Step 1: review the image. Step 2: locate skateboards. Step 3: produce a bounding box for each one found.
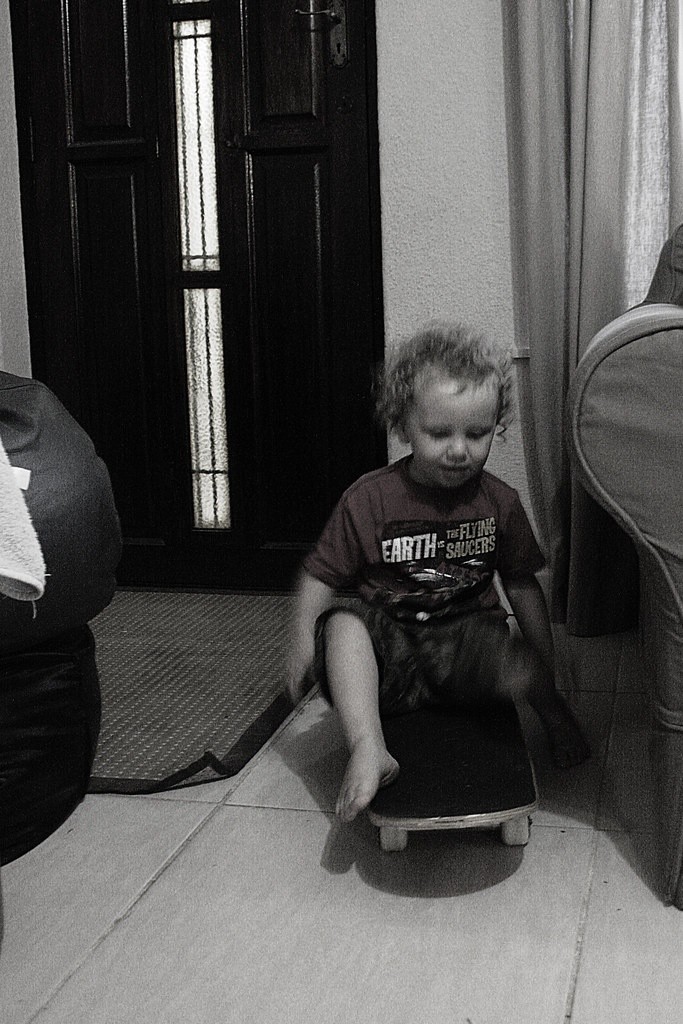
[365,677,543,858]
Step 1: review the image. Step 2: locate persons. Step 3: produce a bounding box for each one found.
[287,323,593,824]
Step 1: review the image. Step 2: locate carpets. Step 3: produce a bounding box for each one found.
[78,590,317,795]
